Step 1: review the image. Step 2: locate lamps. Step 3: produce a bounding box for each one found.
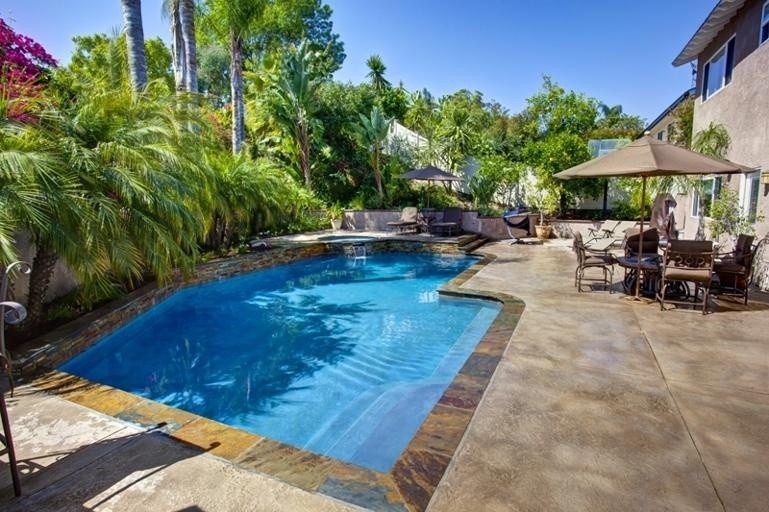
[761,171,769,185]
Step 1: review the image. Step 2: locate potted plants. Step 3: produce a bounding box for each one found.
[331,201,344,229]
[530,177,560,239]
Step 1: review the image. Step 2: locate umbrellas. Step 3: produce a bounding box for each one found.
[552,131,757,301]
[398,166,460,208]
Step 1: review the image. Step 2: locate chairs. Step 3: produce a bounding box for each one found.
[432,205,463,236]
[387,205,417,233]
[0,260,32,498]
[572,219,762,314]
